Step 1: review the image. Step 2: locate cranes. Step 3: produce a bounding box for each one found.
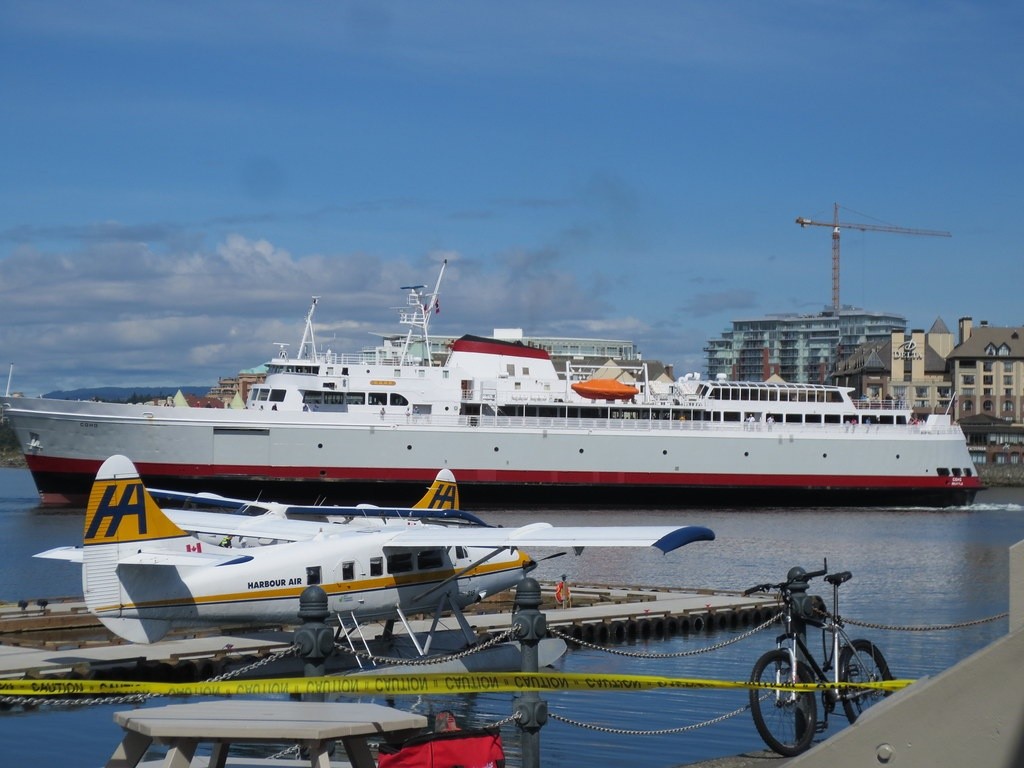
[796,203,952,313]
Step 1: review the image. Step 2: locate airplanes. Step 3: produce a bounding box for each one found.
[32,455,716,645]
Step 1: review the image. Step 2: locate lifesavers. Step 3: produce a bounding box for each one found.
[556,582,571,603]
[0,595,827,713]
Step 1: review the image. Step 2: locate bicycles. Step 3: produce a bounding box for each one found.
[740,557,898,756]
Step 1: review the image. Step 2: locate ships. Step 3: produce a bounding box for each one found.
[1,258,982,512]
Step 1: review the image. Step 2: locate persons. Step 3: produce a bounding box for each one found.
[218,536,233,549]
[679,415,685,429]
[845,392,961,434]
[745,413,776,431]
[92,397,422,425]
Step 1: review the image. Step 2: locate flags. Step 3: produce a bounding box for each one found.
[424,298,440,315]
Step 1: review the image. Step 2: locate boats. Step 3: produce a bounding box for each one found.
[572,378,640,399]
